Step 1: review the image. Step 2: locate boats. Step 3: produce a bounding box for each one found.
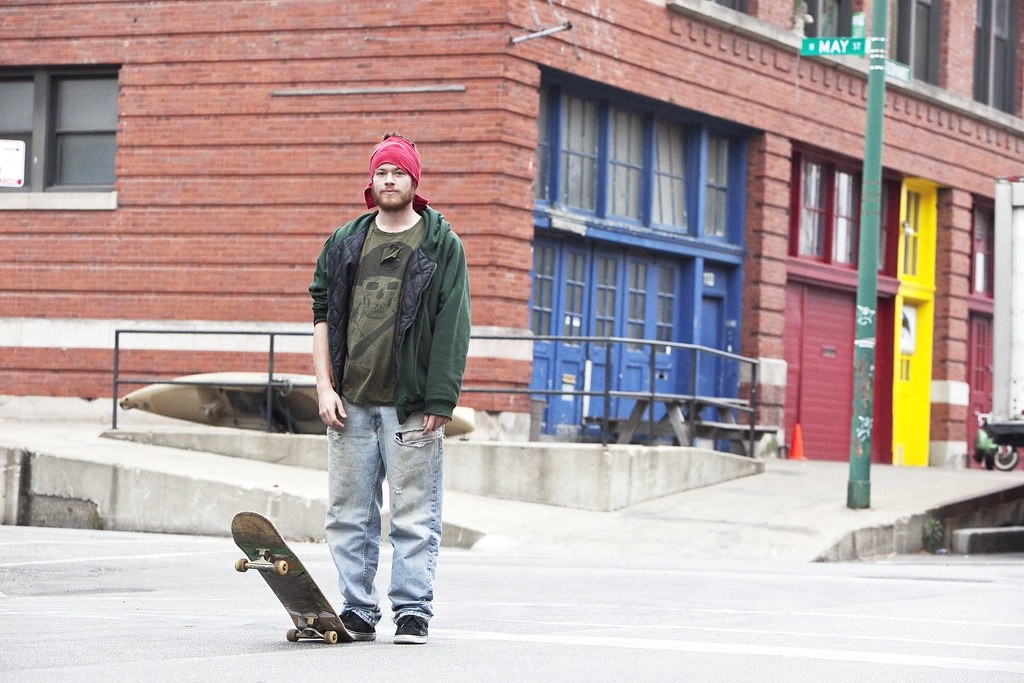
[118,371,476,438]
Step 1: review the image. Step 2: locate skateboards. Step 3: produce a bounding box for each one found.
[230,510,355,644]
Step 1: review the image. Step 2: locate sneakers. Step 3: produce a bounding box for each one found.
[337,610,376,641]
[393,615,429,644]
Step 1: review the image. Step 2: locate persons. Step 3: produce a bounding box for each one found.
[308,134,472,643]
